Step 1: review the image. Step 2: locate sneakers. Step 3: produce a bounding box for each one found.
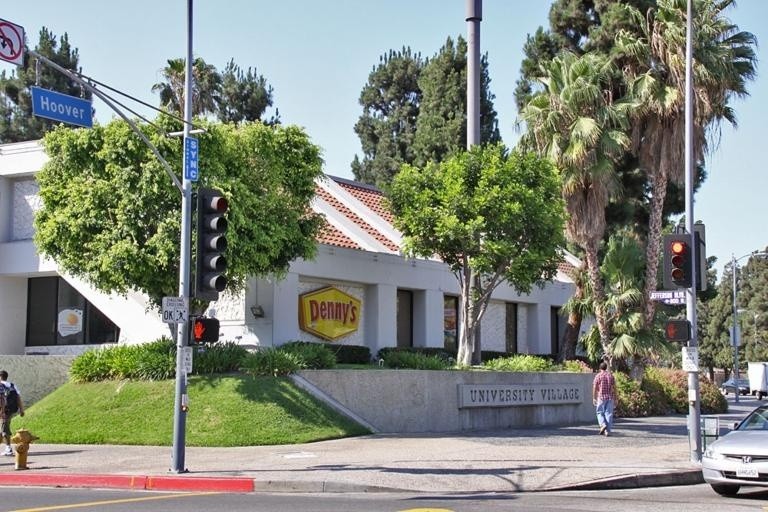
[2,449,13,455]
[599,424,610,436]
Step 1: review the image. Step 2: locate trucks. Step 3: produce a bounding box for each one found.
[748,362,768,400]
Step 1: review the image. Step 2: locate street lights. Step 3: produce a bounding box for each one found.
[730,252,766,402]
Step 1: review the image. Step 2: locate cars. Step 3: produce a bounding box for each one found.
[722,379,750,395]
[701,403,768,497]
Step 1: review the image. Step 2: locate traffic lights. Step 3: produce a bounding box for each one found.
[195,186,228,302]
[188,314,219,345]
[663,233,692,291]
[665,319,690,341]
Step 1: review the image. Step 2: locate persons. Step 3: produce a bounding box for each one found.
[592,362,619,437]
[1,370,25,456]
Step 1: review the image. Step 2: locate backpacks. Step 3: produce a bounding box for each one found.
[0,383,18,415]
